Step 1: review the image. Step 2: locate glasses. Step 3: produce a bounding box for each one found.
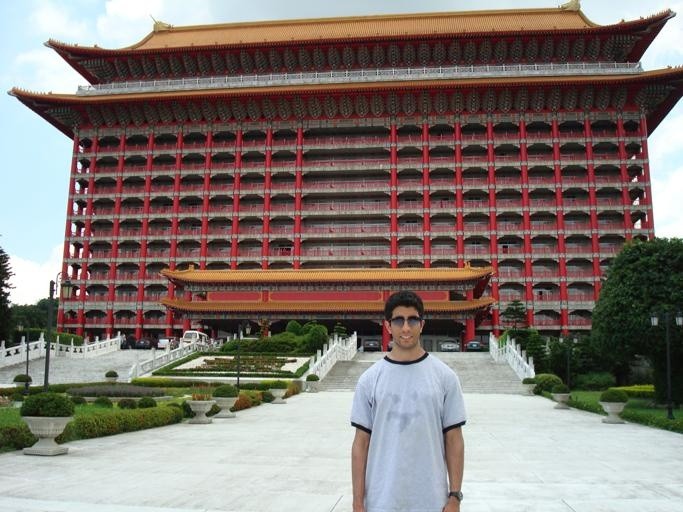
[387,314,422,328]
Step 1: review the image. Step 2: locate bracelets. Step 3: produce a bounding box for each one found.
[447,491,463,501]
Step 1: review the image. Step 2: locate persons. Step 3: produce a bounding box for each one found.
[349,291,465,512]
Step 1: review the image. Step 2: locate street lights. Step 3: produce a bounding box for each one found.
[557,323,581,390]
[17,316,31,398]
[234,320,250,393]
[42,269,71,393]
[650,308,682,419]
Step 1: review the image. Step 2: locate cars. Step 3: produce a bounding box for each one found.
[363,338,379,352]
[386,339,393,350]
[465,340,481,351]
[440,339,459,352]
[120,330,226,353]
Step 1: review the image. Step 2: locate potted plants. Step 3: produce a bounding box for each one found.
[104,370,119,382]
[306,374,320,392]
[550,382,572,409]
[19,389,76,457]
[185,376,241,424]
[598,388,630,424]
[13,374,34,388]
